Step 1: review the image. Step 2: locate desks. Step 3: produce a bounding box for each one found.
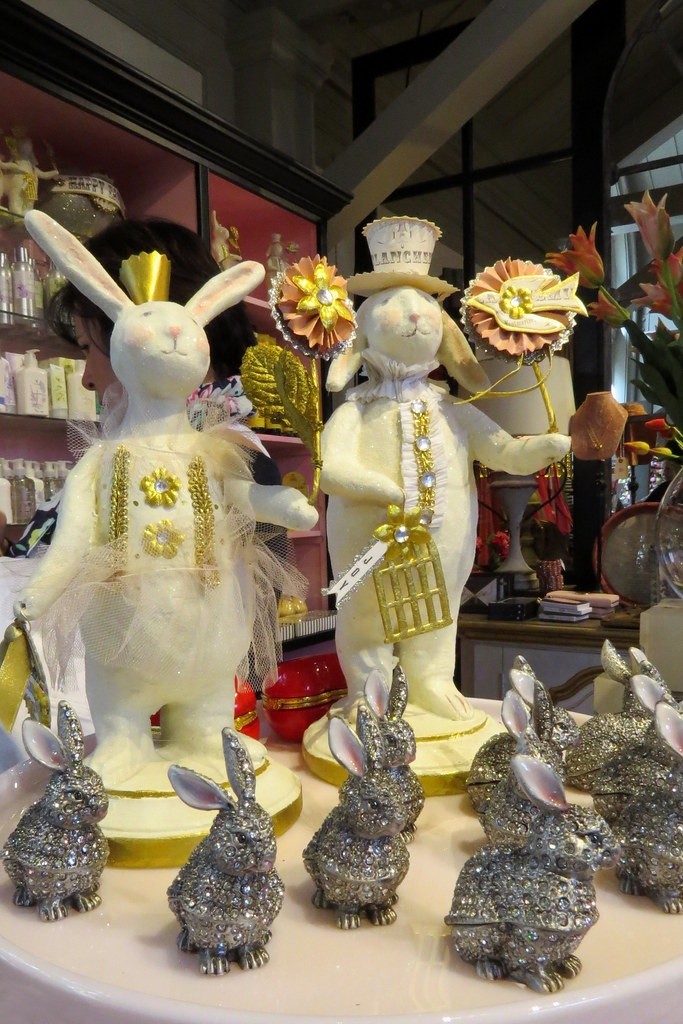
[456,613,641,716]
[0,697,683,1024]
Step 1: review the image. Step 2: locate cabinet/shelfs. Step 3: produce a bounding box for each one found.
[0,0,355,611]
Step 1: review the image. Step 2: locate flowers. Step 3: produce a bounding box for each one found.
[544,190,683,443]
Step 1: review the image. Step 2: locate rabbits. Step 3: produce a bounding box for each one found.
[443,651,625,996]
[1,698,111,923]
[167,726,286,976]
[300,663,426,932]
[559,636,683,917]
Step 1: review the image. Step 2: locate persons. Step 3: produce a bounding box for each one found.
[0,217,288,701]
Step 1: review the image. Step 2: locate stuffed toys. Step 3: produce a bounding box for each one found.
[24,210,571,786]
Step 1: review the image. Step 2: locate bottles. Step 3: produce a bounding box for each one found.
[66,360,96,421]
[0,246,68,339]
